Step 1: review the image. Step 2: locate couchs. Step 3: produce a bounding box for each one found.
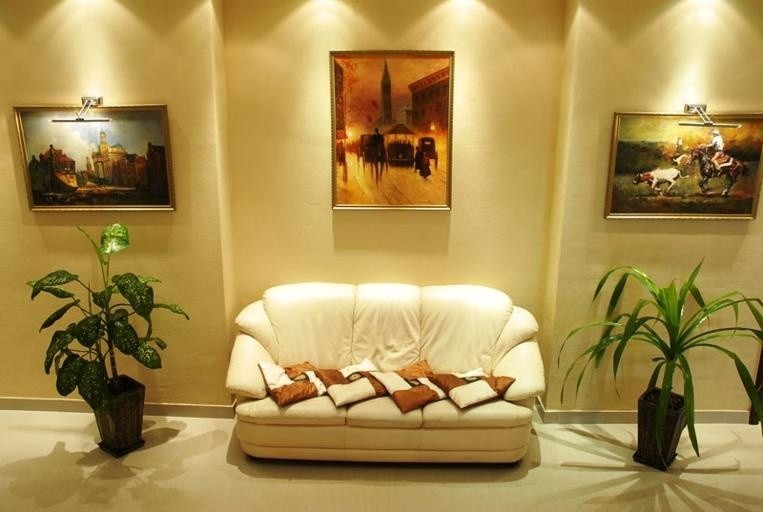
[226,281,546,466]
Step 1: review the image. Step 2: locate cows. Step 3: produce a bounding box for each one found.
[632,167,690,196]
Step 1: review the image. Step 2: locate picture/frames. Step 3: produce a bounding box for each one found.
[329,49,455,211]
[12,104,175,212]
[603,111,763,221]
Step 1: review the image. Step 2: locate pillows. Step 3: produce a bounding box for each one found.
[258,356,516,412]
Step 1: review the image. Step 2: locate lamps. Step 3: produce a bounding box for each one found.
[678,103,741,128]
[52,97,112,122]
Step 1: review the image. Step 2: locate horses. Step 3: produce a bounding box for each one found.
[368,141,385,187]
[687,147,750,198]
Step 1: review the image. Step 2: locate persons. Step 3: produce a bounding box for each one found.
[704,128,724,178]
[336,126,432,186]
[674,136,690,178]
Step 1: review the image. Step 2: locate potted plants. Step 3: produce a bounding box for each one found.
[557,255,763,472]
[25,223,189,458]
